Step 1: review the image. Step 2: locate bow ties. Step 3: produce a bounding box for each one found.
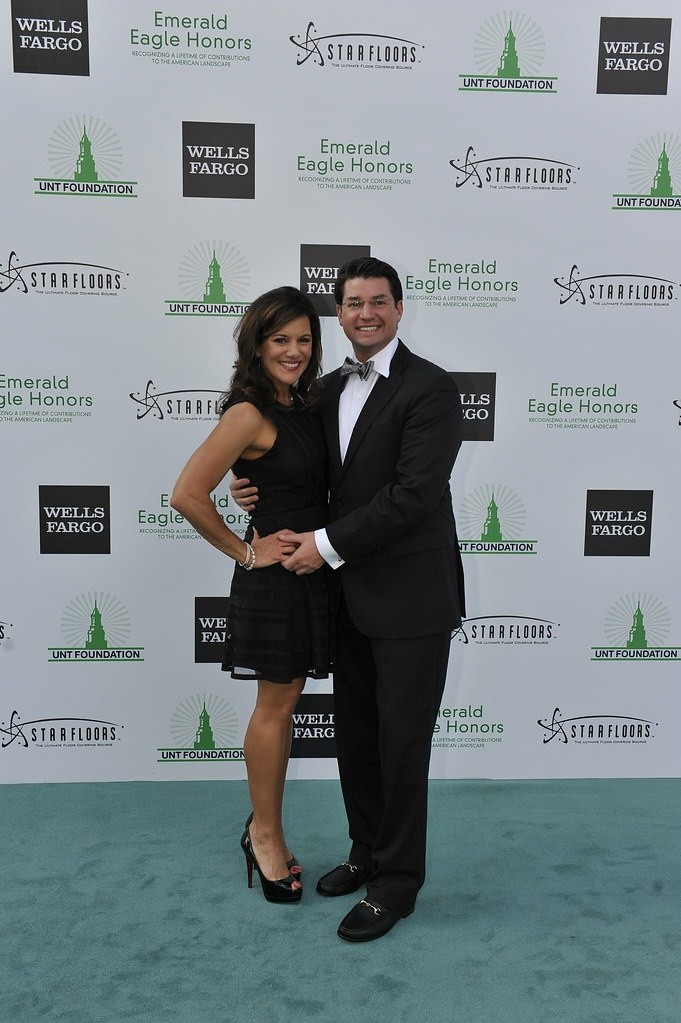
[340,356,374,381]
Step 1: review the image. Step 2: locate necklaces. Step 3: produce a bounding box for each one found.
[288,391,295,401]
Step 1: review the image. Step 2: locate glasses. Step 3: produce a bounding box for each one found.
[340,296,398,311]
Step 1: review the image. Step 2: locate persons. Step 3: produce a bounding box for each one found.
[169,288,339,906]
[231,256,467,943]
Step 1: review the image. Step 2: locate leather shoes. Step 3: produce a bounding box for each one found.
[315,859,416,941]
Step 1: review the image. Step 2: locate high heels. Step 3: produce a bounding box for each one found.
[240,808,304,906]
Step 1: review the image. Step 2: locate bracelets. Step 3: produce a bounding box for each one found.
[243,544,255,571]
[236,542,250,567]
